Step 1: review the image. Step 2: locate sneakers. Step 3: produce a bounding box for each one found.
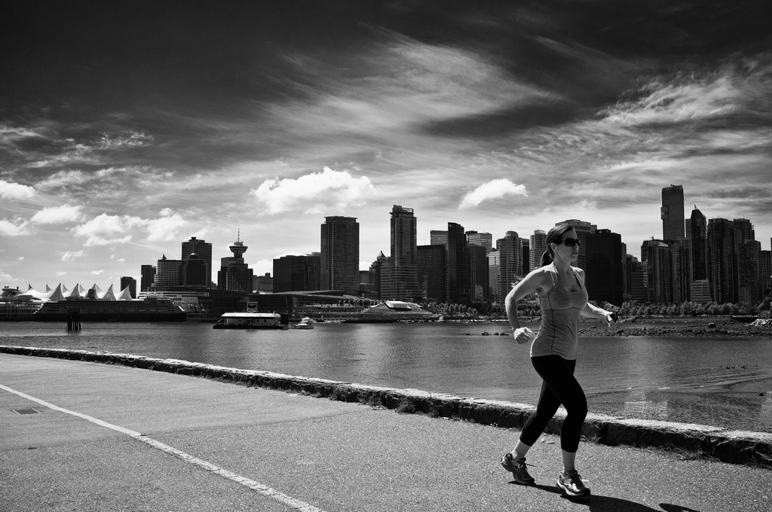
[500,453,534,485]
[556,470,590,496]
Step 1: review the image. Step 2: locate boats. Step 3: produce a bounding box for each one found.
[212,313,284,330]
[296,317,315,330]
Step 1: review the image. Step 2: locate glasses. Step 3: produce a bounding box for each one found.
[561,238,582,247]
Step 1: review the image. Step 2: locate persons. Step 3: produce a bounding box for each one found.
[501,223,617,498]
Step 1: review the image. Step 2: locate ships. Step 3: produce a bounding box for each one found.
[33,295,185,320]
